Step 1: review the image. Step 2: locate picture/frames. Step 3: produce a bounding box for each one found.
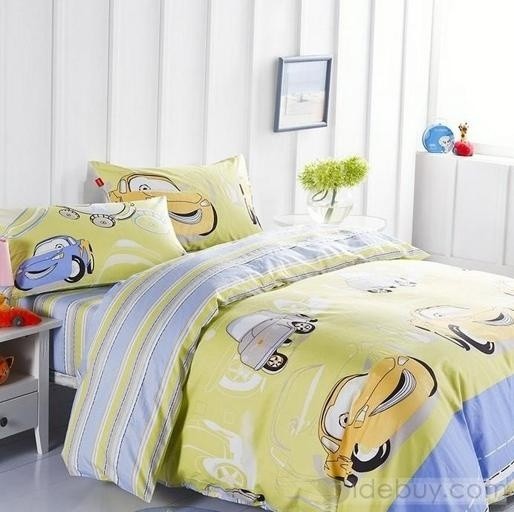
[272,54,331,135]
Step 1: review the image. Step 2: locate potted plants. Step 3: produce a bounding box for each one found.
[296,154,370,227]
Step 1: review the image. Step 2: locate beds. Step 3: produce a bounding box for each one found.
[0,207,513,494]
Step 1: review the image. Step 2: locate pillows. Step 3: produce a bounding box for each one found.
[0,154,267,300]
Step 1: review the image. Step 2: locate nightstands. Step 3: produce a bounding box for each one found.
[0,315,62,456]
